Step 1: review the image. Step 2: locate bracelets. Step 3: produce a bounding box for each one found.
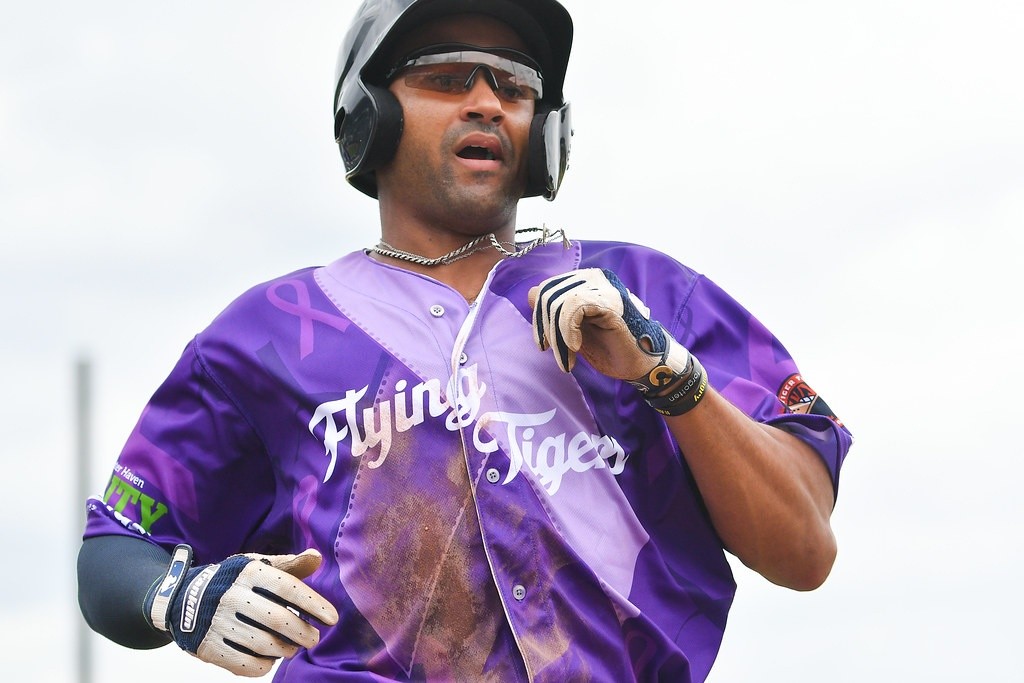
[645,354,708,416]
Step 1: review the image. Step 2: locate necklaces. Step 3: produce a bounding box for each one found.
[374,225,570,265]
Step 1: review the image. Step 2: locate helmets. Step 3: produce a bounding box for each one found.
[331,0,573,201]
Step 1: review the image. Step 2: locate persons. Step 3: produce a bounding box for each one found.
[76,1,853,683]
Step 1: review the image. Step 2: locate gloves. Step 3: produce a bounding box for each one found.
[527,266,693,397]
[139,540,341,677]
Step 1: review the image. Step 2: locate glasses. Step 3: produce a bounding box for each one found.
[380,42,547,101]
